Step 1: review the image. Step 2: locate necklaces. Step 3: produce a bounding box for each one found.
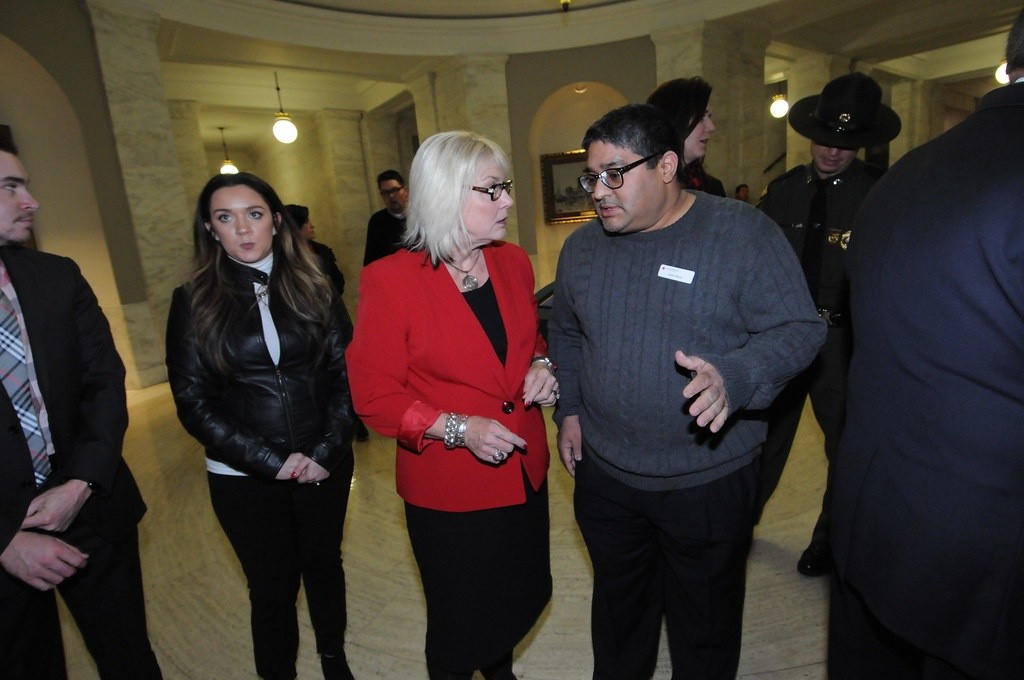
[440,248,481,292]
[256,288,267,299]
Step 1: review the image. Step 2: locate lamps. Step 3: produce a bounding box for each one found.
[270,70,299,144]
[995,60,1011,84]
[560,0,571,12]
[216,125,239,175]
[769,82,790,118]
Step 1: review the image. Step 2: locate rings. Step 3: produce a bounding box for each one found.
[493,450,505,462]
[551,390,560,399]
[314,481,320,485]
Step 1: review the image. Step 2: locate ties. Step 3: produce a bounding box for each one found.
[0,291,51,487]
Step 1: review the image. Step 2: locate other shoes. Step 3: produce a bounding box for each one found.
[355,418,369,441]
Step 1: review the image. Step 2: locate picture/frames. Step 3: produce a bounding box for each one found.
[538,147,602,227]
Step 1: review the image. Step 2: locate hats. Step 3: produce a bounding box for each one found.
[788,73,902,150]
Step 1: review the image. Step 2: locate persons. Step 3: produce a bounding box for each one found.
[647,75,728,201]
[734,183,749,203]
[342,131,561,680]
[164,172,363,680]
[823,5,1024,680]
[745,72,902,580]
[549,102,827,680]
[364,169,423,269]
[282,204,370,446]
[1,124,164,680]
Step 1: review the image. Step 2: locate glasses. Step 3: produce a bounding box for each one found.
[463,179,513,202]
[577,151,664,194]
[379,185,404,198]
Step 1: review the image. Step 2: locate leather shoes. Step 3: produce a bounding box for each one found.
[798,545,836,577]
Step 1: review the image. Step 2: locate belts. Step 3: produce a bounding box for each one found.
[815,309,852,325]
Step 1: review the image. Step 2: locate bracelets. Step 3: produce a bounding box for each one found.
[444,413,472,449]
[87,481,97,492]
[530,355,555,375]
